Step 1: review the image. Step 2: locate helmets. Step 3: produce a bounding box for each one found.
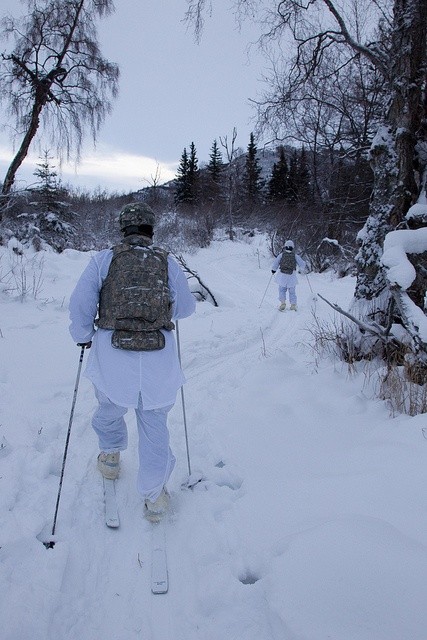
[284,240,295,250]
[116,202,155,233]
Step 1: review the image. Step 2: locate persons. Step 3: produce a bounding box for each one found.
[271,238,302,311]
[65,199,196,515]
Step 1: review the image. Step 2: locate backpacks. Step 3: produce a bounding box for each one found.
[279,250,296,275]
[89,244,174,351]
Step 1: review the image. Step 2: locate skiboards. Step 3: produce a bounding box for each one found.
[102,477,170,595]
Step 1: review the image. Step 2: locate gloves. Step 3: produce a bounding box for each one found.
[271,269,276,274]
[76,338,92,352]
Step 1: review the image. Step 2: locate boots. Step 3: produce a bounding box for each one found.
[96,451,121,482]
[144,487,173,521]
[290,305,298,312]
[278,301,287,311]
